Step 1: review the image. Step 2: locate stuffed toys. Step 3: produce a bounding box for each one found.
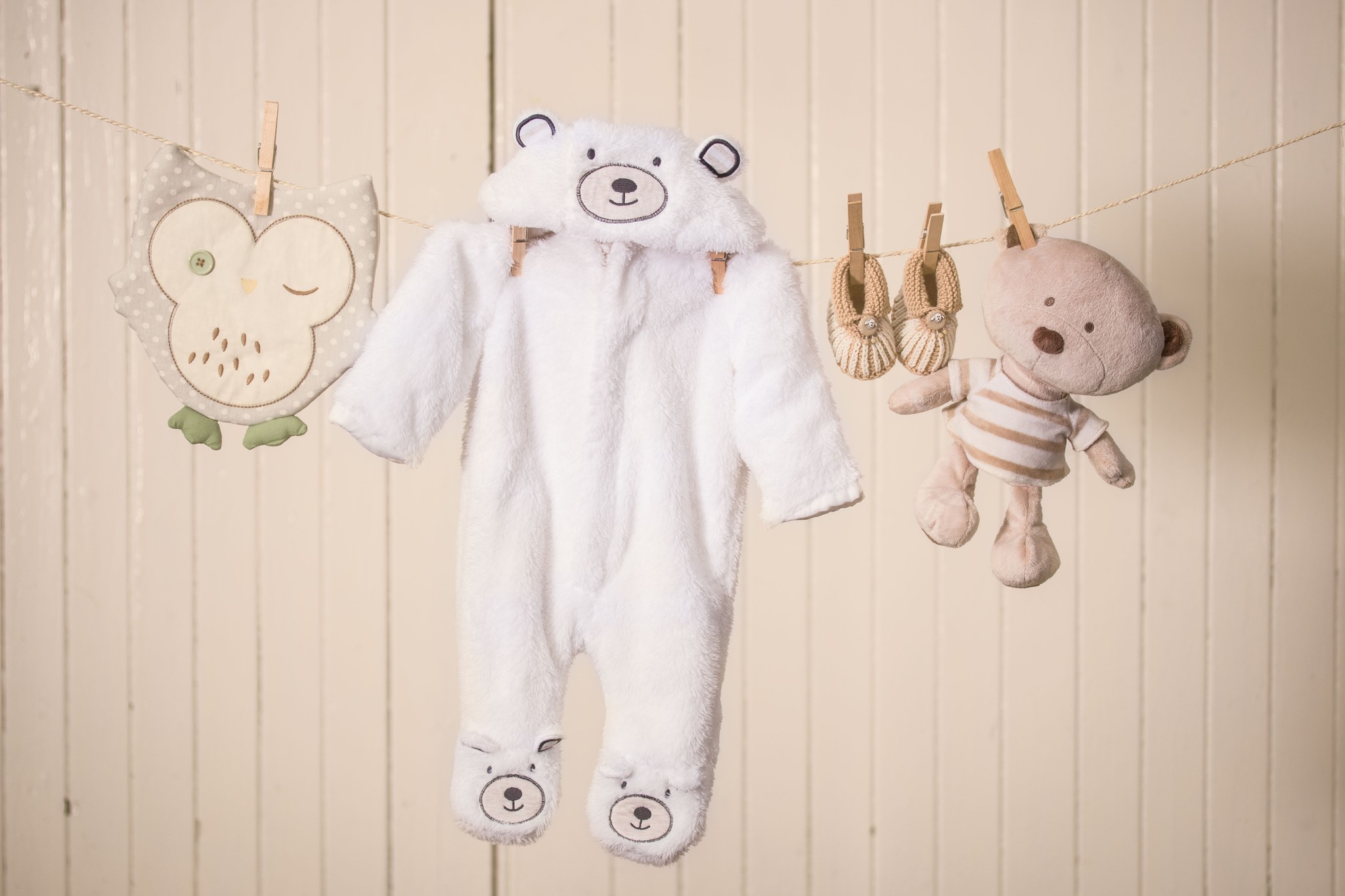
[889,219,1194,592]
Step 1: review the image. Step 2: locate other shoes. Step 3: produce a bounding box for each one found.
[891,249,964,376]
[826,253,897,381]
